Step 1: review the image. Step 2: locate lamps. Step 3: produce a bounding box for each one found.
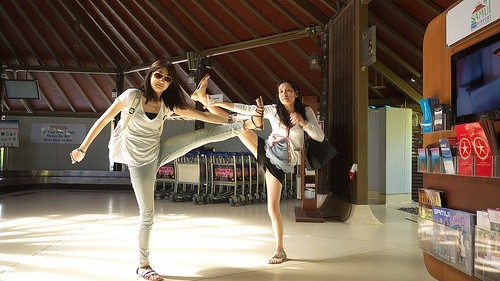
[188,71,195,85]
[310,51,320,70]
[112,88,117,98]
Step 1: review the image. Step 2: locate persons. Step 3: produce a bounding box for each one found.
[69,59,264,281]
[190,72,325,264]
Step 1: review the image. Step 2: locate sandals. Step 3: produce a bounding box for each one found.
[267,251,287,264]
[251,96,265,131]
[191,74,211,103]
[136,265,164,281]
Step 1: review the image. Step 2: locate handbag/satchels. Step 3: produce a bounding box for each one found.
[301,104,338,171]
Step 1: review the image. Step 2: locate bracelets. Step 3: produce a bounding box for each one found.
[227,116,232,123]
[77,147,86,154]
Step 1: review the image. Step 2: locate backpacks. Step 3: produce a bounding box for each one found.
[108,88,168,164]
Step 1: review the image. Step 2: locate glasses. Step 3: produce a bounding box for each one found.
[152,71,174,84]
